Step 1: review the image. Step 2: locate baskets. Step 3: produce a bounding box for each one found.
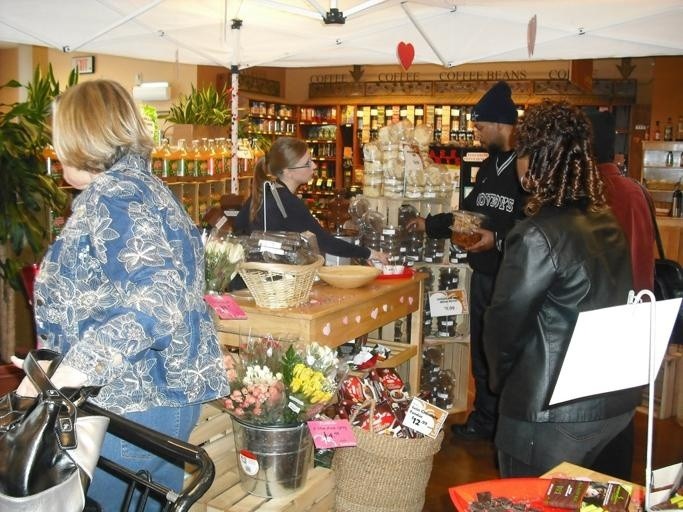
[236,255,326,311]
[331,396,445,511]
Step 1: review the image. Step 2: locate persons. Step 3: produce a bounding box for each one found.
[482,99,643,478]
[225,135,391,292]
[32,79,230,508]
[405,81,530,443]
[579,103,657,482]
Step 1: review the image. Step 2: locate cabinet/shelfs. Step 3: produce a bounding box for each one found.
[217,89,613,224]
[641,140,683,267]
[47,157,276,242]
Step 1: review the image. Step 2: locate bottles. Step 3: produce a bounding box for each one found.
[676,115,682,141]
[149,138,265,181]
[665,117,672,140]
[654,120,660,142]
[181,192,220,222]
[248,101,293,136]
[671,187,682,217]
[435,130,474,148]
[307,127,336,158]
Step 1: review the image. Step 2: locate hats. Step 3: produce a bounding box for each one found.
[583,107,616,162]
[470,80,518,124]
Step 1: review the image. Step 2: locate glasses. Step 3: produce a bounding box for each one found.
[278,152,312,174]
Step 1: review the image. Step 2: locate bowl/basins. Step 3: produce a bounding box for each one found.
[316,265,378,289]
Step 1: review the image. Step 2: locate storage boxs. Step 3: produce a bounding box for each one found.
[173,124,227,148]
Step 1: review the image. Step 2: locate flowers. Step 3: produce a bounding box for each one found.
[156,80,241,139]
[205,239,245,293]
[223,337,339,427]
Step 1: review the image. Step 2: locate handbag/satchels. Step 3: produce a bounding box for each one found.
[0,348,110,512]
[632,175,683,345]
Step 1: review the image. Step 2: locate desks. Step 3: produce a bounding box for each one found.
[214,272,429,467]
[538,462,646,512]
